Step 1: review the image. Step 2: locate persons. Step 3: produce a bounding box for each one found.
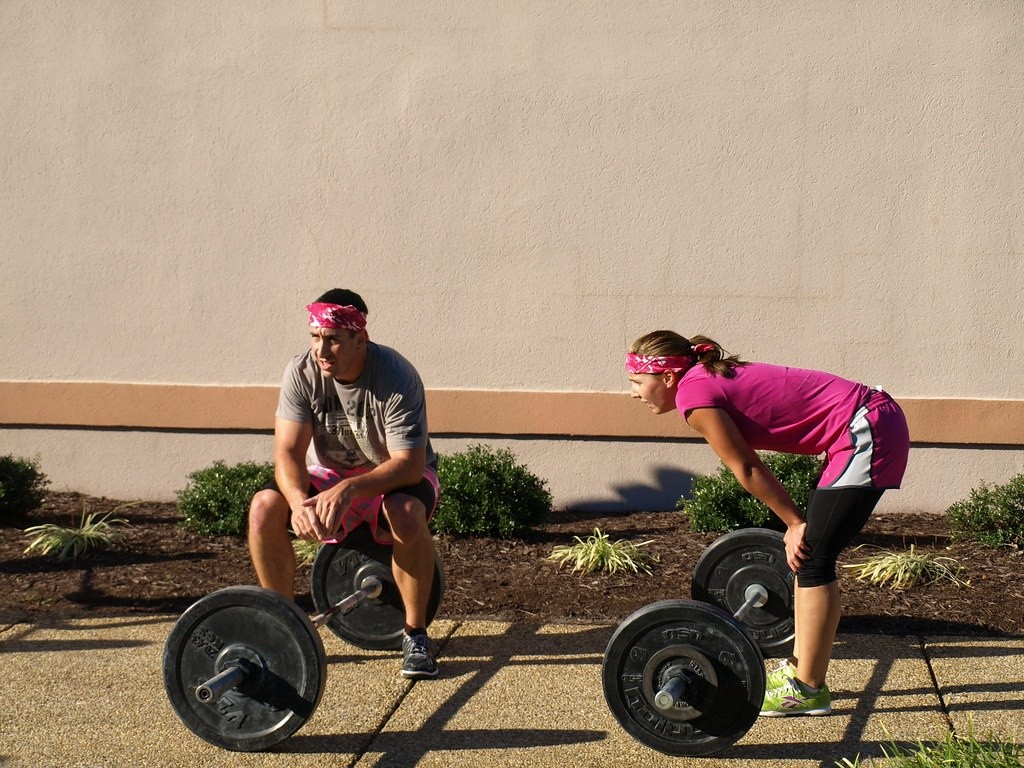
[248,286,444,680]
[626,329,912,717]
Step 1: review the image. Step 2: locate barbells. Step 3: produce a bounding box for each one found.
[158,521,448,755]
[598,522,816,760]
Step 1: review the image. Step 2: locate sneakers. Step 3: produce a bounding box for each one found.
[758,678,831,717]
[401,635,438,679]
[765,658,798,691]
